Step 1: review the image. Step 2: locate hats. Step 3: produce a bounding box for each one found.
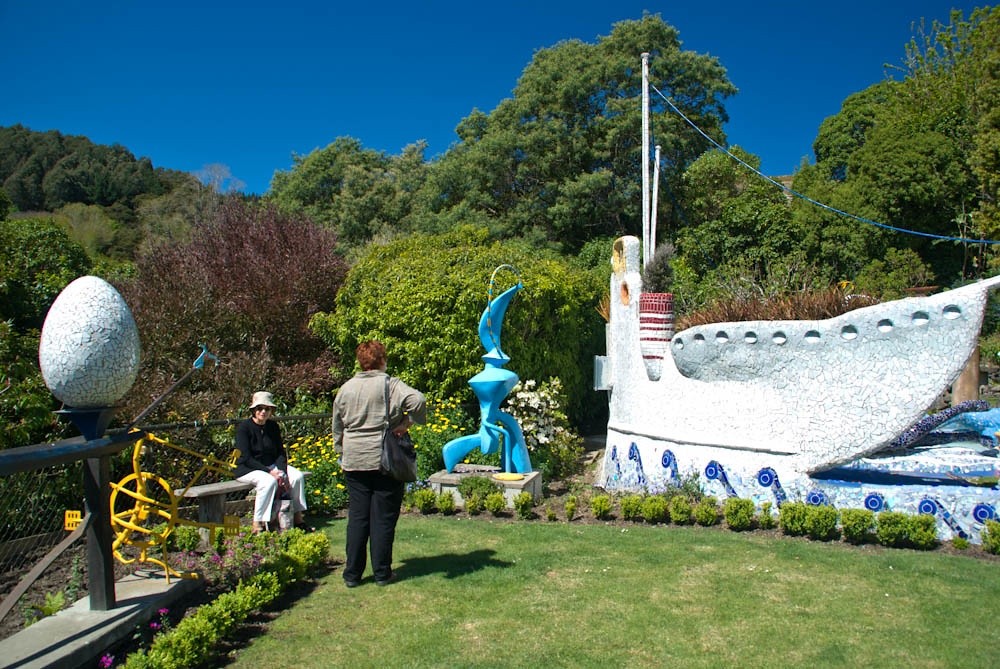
[249,391,278,409]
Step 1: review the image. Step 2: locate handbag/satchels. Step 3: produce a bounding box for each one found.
[381,376,417,482]
[268,476,293,532]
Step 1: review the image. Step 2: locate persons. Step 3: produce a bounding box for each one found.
[233,391,312,534]
[332,340,426,589]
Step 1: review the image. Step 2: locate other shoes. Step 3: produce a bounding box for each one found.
[375,573,400,586]
[294,522,315,533]
[344,578,362,588]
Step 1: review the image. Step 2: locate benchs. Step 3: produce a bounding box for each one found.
[174,472,311,523]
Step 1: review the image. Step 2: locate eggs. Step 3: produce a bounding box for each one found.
[39,275,142,409]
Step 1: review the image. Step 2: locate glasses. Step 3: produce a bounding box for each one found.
[256,407,271,412]
